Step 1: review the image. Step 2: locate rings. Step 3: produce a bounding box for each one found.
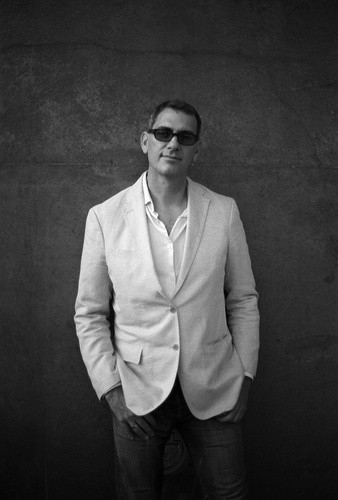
[131,424,137,430]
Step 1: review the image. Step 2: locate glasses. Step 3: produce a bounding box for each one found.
[147,127,200,146]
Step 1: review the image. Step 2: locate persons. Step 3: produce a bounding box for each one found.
[73,99,259,500]
